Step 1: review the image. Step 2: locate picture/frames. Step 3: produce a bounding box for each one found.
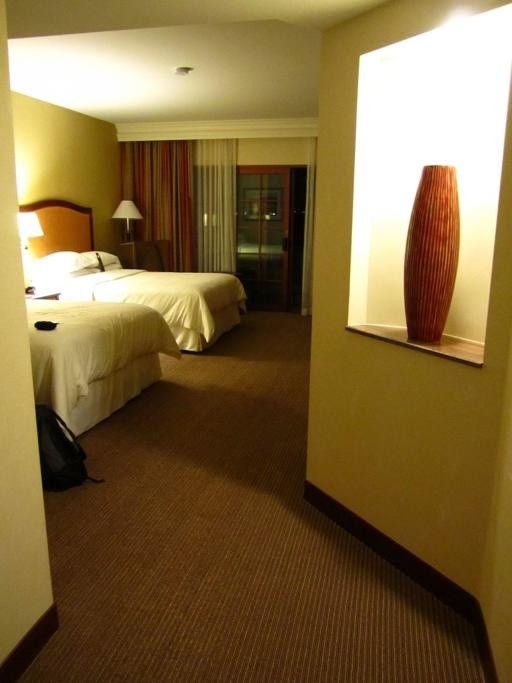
[243,187,284,223]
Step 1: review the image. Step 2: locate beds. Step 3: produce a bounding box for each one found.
[24,299,180,443]
[20,201,246,352]
[237,235,284,263]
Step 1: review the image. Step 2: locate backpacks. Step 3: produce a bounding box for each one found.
[35,401,105,492]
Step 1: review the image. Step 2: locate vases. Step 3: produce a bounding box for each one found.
[405,166,459,346]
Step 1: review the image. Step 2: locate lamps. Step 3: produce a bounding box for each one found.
[111,200,143,241]
[18,212,44,238]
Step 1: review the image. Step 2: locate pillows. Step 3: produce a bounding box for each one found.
[39,251,123,275]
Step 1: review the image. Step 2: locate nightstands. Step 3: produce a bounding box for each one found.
[24,288,60,300]
